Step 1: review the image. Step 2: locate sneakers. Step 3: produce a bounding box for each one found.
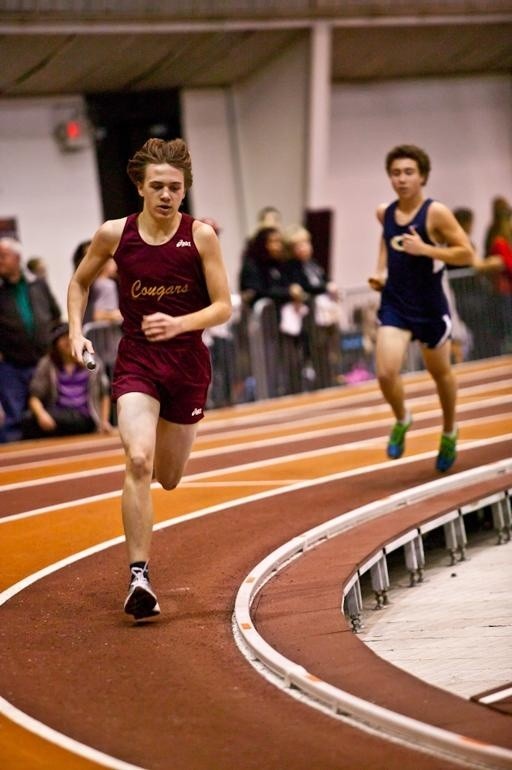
[434,425,460,473]
[124,567,162,622]
[386,415,412,461]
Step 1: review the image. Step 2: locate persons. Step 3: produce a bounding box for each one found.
[369,144,472,473]
[1,236,124,441]
[198,206,345,408]
[66,136,234,618]
[445,208,479,295]
[472,204,512,299]
